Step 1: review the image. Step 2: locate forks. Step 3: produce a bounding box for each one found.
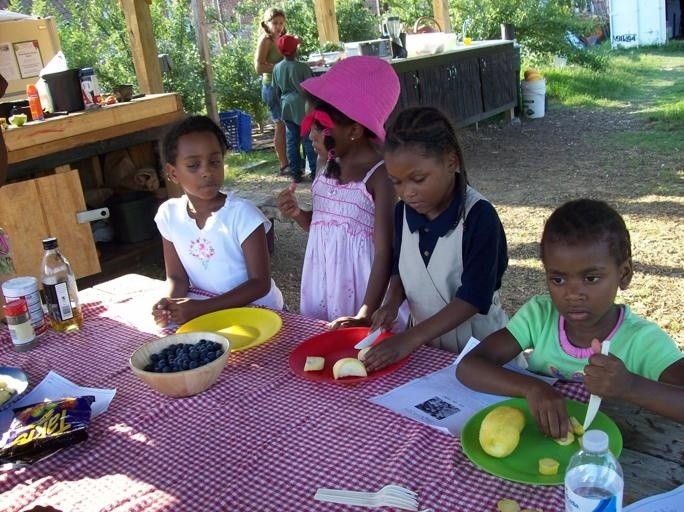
[313,485,419,512]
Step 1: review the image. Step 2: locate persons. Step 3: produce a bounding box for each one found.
[276,56,401,331]
[152,115,287,328]
[363,108,528,374]
[456,199,684,439]
[254,7,292,175]
[271,35,316,183]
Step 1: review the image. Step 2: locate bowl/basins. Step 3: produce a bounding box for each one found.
[128,332,231,398]
[8,113,27,126]
[113,85,132,102]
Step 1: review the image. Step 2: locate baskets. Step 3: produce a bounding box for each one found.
[219,109,253,153]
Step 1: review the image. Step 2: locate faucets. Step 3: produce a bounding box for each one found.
[411,16,442,33]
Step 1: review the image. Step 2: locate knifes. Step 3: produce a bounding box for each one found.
[582,341,609,430]
[354,325,386,350]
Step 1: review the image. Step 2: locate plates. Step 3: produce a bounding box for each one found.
[289,327,415,386]
[0,366,31,411]
[175,306,284,353]
[462,393,624,486]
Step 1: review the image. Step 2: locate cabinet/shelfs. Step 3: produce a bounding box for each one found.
[0,120,187,304]
[313,46,522,131]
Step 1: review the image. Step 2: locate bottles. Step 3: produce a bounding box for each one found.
[80,67,100,107]
[565,431,624,511]
[464,35,471,47]
[26,83,44,121]
[0,237,84,351]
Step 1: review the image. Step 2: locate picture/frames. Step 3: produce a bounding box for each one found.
[12,40,45,79]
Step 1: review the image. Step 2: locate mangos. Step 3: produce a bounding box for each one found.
[478,404,526,459]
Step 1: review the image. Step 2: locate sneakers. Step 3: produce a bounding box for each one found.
[280,164,292,176]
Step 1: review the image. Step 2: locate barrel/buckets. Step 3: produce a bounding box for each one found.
[41,68,86,113]
[520,77,547,120]
[103,190,157,244]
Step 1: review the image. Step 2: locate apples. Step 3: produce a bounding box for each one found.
[303,356,326,372]
[333,357,367,379]
[358,345,372,361]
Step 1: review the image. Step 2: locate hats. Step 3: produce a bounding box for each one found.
[299,55,401,145]
[278,35,300,56]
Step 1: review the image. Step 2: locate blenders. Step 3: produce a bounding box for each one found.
[382,16,407,58]
